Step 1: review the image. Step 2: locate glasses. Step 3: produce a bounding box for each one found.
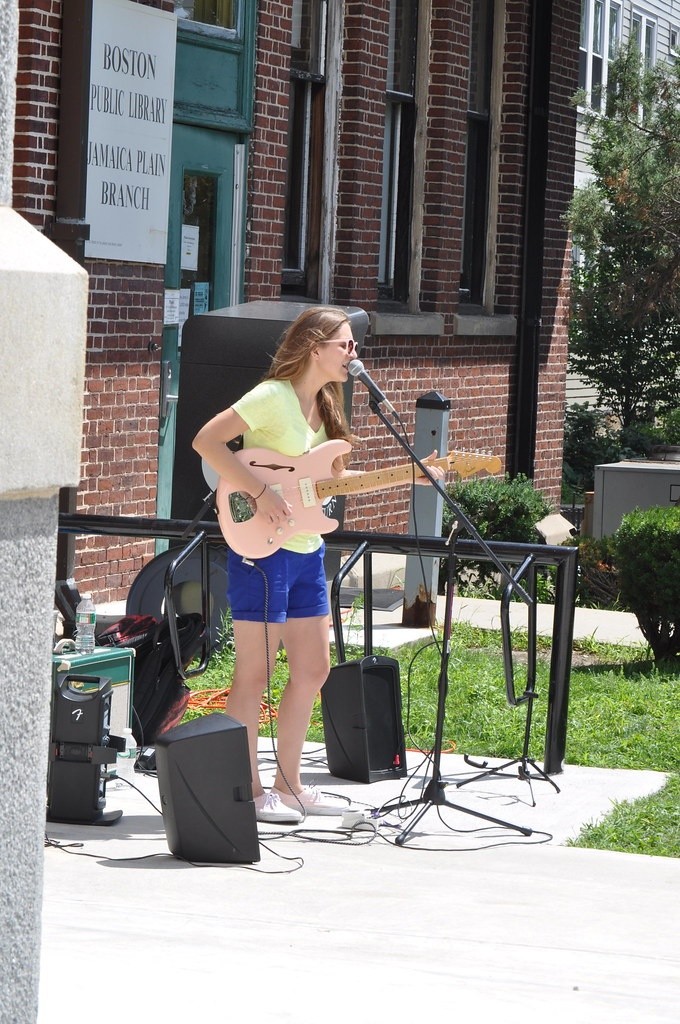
[317,339,358,355]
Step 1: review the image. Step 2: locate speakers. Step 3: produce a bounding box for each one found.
[154,712,261,862]
[320,654,407,783]
[49,673,125,826]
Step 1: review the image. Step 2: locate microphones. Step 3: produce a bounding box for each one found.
[349,359,399,418]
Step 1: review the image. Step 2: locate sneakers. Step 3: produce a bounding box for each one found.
[270,786,350,816]
[252,792,302,822]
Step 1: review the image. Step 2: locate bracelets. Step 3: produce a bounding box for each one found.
[253,484,267,499]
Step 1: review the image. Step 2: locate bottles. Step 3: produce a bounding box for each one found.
[74,592,97,655]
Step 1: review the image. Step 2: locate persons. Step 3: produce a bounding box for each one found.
[193,307,446,824]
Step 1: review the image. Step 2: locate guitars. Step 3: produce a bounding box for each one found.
[215,437,502,559]
